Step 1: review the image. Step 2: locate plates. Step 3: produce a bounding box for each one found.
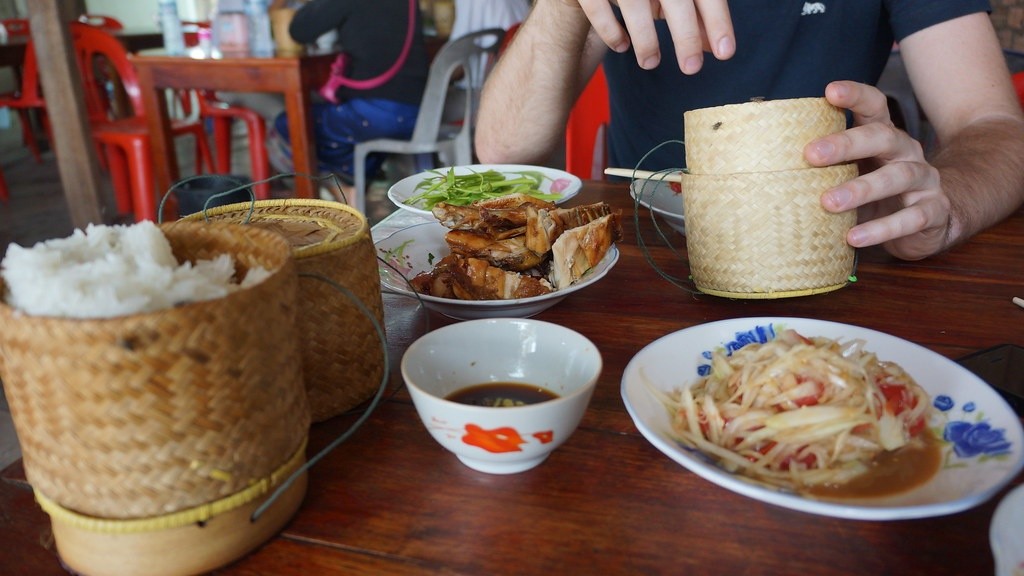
[620,316,1024,521]
[989,488,1024,576]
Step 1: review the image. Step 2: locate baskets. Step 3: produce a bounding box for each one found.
[681,97,860,298]
[174,198,388,422]
[0,222,310,519]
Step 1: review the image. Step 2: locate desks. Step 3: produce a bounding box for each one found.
[131,49,339,222]
[1,33,163,93]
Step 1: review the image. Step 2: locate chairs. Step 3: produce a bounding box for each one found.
[0,18,612,216]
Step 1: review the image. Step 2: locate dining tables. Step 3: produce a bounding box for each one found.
[2,182,1024,576]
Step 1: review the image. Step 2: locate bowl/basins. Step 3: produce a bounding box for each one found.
[629,168,686,236]
[401,319,604,475]
[388,164,581,222]
[375,223,618,322]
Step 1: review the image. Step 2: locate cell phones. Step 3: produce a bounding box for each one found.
[953,343,1024,416]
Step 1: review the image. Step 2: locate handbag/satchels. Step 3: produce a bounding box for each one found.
[320,53,351,105]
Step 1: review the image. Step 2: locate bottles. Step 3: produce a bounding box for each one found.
[244,1,273,55]
[158,0,184,56]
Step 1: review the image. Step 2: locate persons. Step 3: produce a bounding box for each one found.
[265,0,533,225]
[474,0,1024,259]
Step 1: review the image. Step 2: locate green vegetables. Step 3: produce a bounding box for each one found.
[404,169,560,208]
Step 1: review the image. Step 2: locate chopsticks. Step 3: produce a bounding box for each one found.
[604,167,680,182]
